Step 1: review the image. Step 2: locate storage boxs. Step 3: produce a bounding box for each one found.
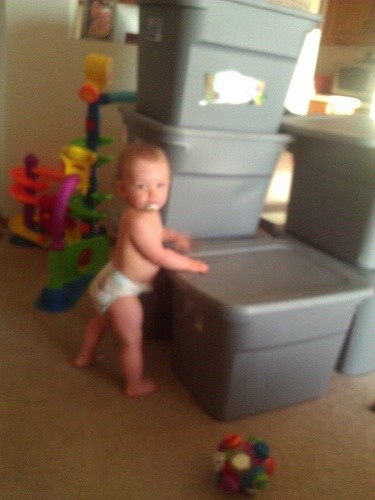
[116,0,375,423]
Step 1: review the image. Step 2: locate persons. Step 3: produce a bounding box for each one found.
[74,140,209,398]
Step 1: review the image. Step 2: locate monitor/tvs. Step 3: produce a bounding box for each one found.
[331,62,375,100]
